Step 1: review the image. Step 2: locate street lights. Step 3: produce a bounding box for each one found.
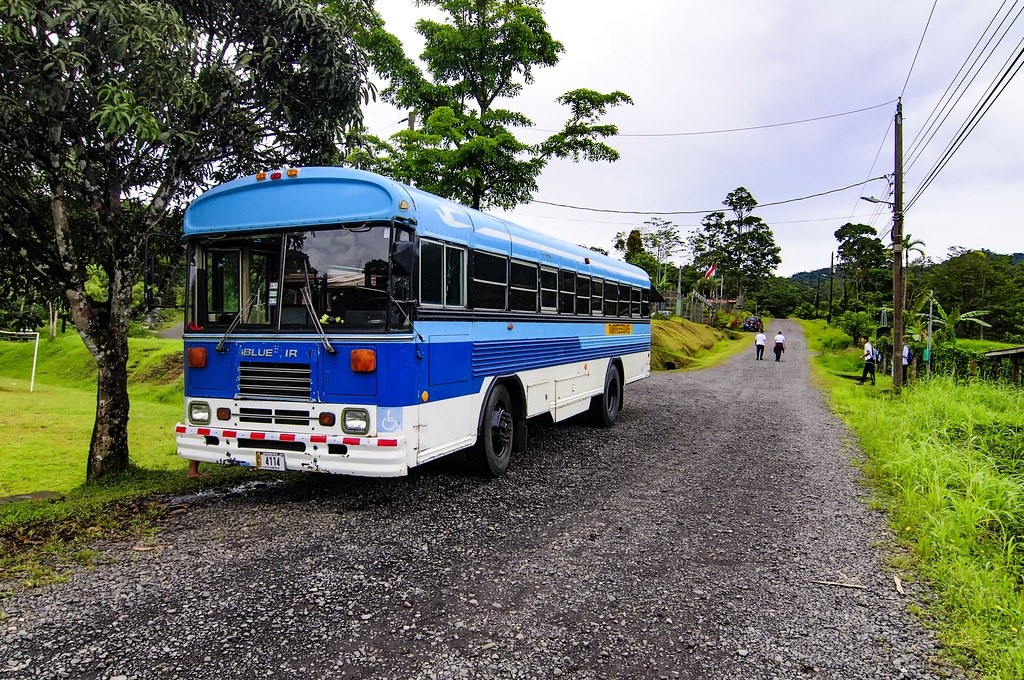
[820,274,833,324]
[860,196,905,393]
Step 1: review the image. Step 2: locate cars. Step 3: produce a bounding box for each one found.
[651,311,676,318]
[744,316,759,330]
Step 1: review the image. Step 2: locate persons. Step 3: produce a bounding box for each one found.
[186,460,207,478]
[755,329,766,360]
[773,331,786,362]
[731,317,764,333]
[901,342,909,386]
[855,336,875,386]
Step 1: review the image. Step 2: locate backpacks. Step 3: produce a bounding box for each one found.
[902,346,913,365]
[870,344,881,363]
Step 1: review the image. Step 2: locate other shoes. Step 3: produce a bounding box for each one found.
[775,359,780,362]
[854,381,864,385]
[756,358,759,360]
[869,382,875,386]
[760,359,763,360]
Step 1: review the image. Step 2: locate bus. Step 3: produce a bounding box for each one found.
[143,167,650,478]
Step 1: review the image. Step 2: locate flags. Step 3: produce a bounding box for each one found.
[705,261,716,280]
